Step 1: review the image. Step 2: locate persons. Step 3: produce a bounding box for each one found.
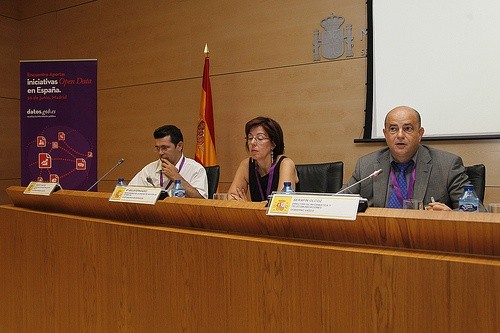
[124,125,209,200]
[333,105,487,213]
[223,117,300,204]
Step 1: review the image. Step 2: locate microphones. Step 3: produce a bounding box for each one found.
[336,168,383,195]
[87,158,124,192]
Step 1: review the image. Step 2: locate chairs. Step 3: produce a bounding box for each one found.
[205,165,220,199]
[465,164,486,204]
[295,162,344,193]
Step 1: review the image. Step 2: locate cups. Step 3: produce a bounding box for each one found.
[488,203,500,213]
[403,199,424,210]
[213,193,230,201]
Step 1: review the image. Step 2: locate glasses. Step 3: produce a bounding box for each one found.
[244,133,271,142]
[152,145,167,153]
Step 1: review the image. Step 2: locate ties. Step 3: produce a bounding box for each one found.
[388,164,409,208]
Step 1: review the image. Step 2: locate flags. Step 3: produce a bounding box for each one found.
[194,56,221,200]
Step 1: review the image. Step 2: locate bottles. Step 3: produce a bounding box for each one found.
[280,182,294,192]
[117,177,126,186]
[171,180,186,198]
[458,185,479,212]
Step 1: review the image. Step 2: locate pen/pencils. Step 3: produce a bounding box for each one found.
[431,197,435,203]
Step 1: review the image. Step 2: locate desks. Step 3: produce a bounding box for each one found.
[0,186,499,333]
